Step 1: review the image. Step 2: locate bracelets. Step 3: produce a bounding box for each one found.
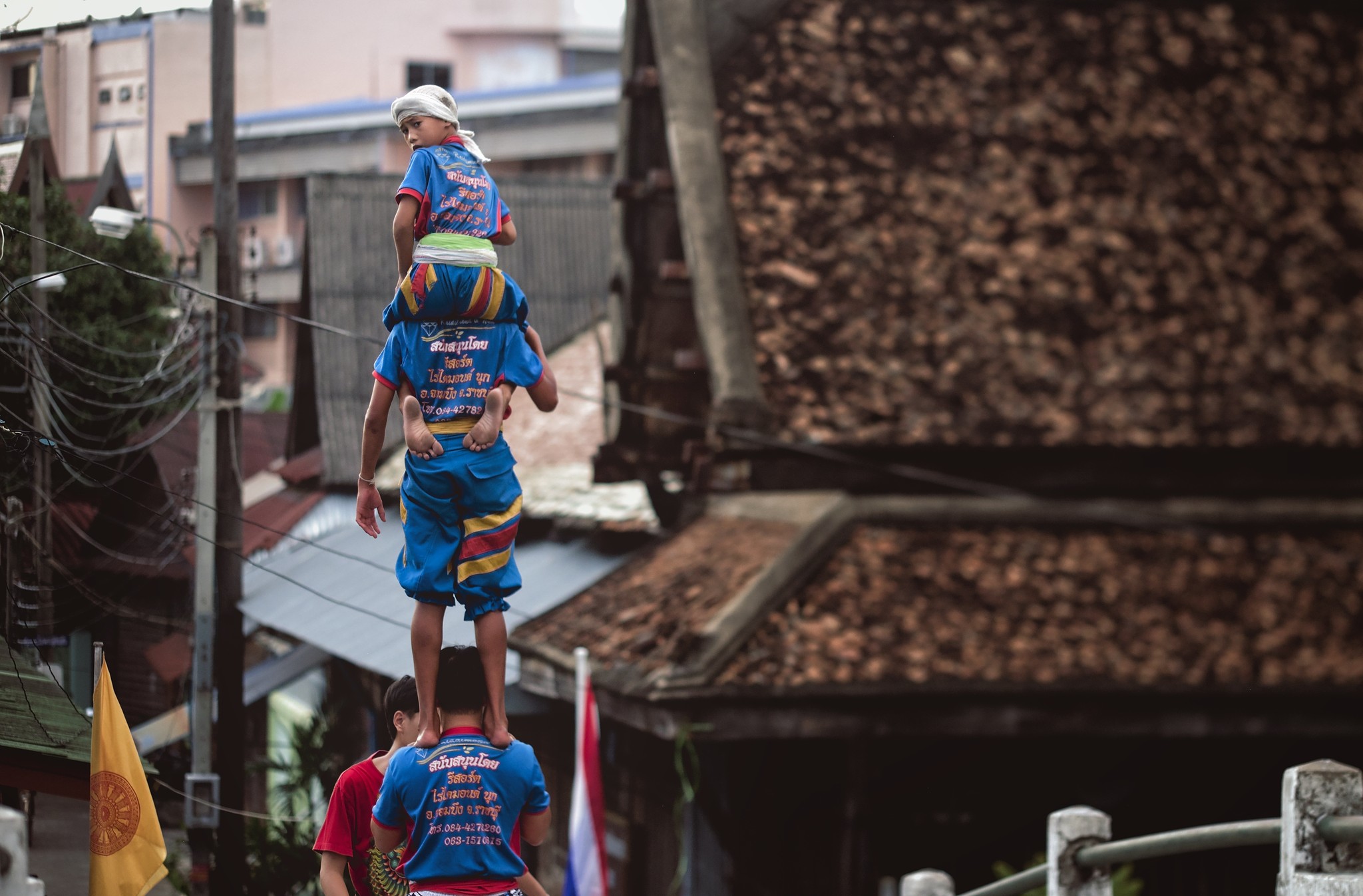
[357,473,376,486]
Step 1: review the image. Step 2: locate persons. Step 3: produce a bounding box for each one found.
[357,320,562,745]
[378,81,538,461]
[369,643,554,896]
[311,672,552,896]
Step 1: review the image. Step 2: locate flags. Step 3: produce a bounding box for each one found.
[86,659,170,895]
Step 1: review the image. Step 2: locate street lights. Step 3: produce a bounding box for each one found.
[90,203,225,896]
[0,270,66,331]
